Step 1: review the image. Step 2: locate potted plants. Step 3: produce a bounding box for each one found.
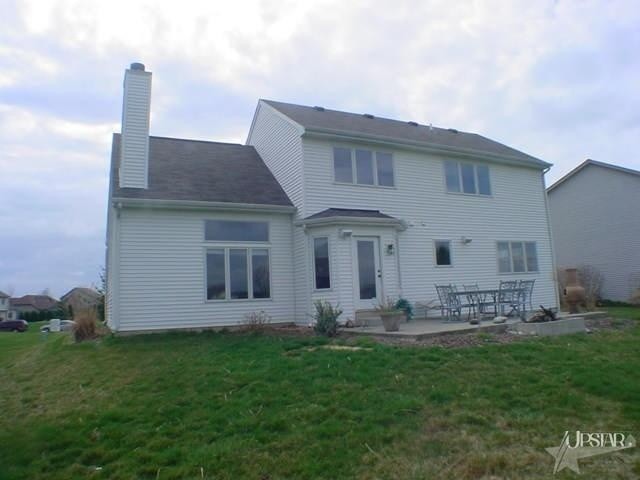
[372,296,405,337]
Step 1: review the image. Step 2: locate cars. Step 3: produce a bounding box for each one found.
[40,319,75,332]
[0,319,28,332]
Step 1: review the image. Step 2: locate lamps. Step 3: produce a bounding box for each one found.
[463,235,474,244]
[339,227,353,239]
[386,243,396,257]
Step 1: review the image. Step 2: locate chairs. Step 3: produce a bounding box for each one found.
[431,277,536,323]
